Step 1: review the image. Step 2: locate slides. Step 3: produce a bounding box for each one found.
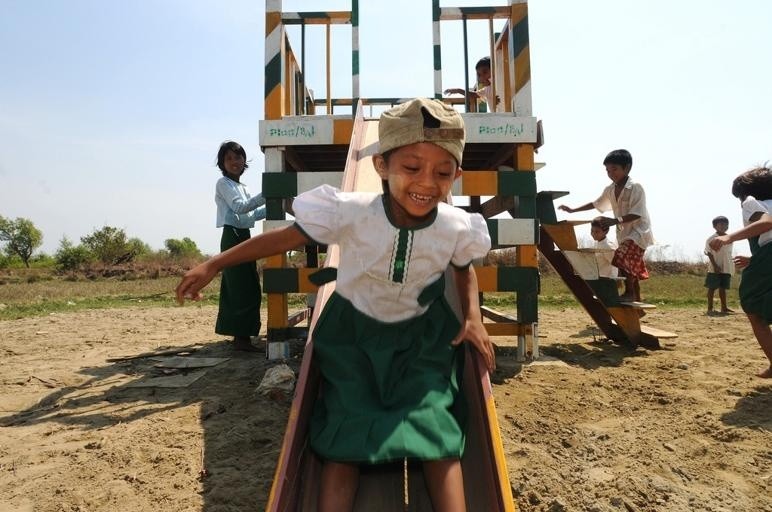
[263,98,514,512]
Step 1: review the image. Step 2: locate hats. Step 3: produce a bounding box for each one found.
[377,99,467,167]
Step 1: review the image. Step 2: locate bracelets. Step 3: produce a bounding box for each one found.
[617,217,624,225]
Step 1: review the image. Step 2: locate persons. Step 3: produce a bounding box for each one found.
[557,149,654,318]
[708,168,772,378]
[214,141,268,353]
[704,215,736,314]
[590,216,623,327]
[176,95,497,512]
[444,57,493,113]
